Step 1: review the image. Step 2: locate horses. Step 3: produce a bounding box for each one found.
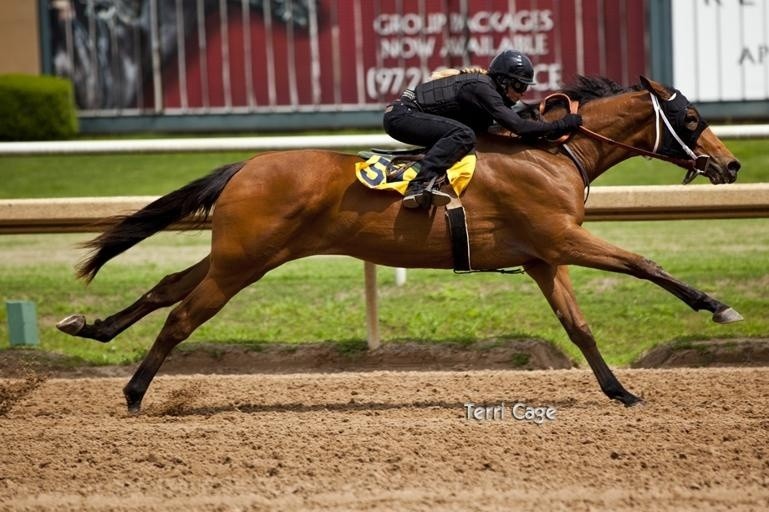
[56,71,744,412]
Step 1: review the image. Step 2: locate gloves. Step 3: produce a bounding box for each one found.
[557,114,582,129]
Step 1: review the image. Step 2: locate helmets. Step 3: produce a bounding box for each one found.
[487,51,537,85]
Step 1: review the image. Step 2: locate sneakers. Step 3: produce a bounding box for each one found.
[403,190,452,208]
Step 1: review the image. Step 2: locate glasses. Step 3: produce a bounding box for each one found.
[497,75,527,93]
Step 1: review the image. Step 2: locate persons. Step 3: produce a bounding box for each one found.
[382,52,582,210]
